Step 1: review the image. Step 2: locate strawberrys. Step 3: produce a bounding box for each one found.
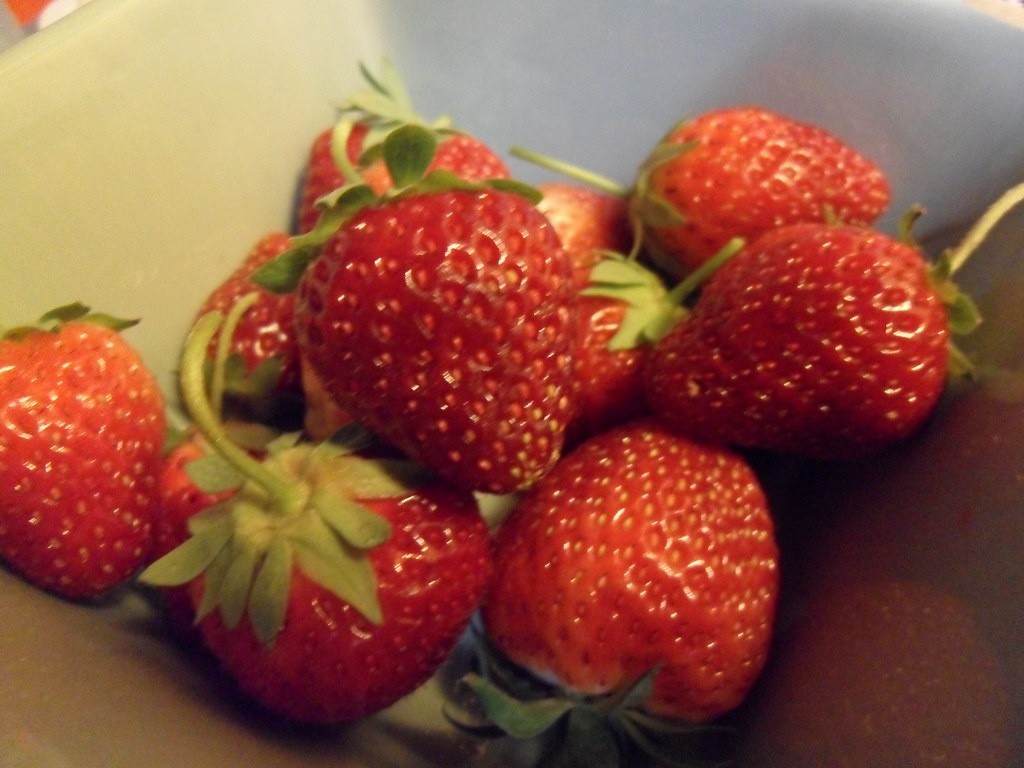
[0,60,984,763]
